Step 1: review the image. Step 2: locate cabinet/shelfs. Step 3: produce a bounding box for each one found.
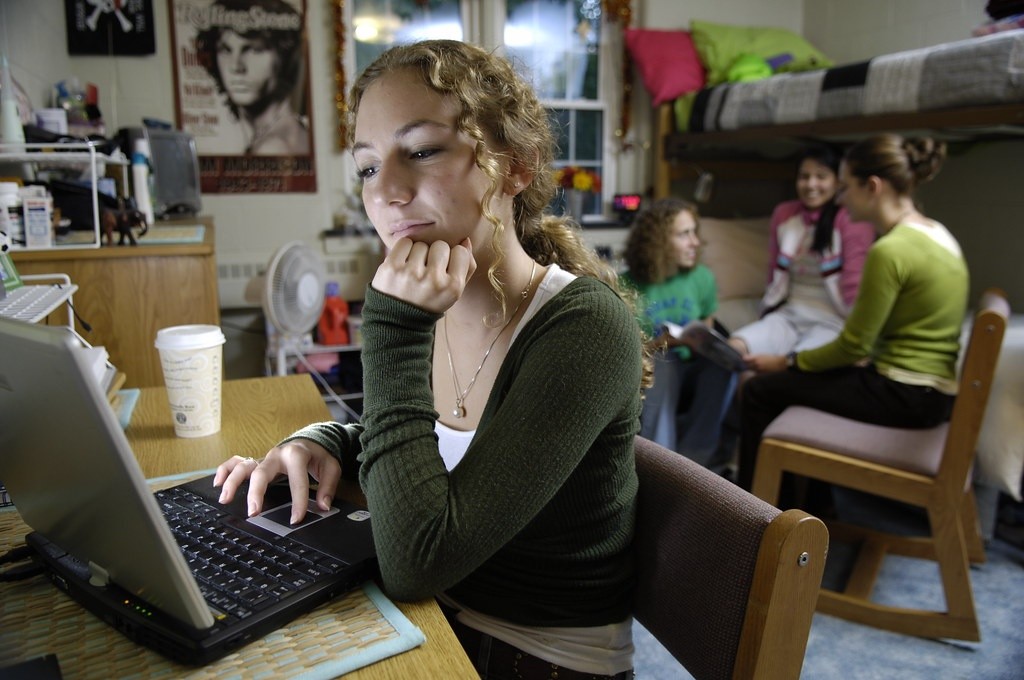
[263,343,364,401]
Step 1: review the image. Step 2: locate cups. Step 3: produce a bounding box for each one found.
[154,324,226,439]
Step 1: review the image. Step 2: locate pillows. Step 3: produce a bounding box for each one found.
[696,214,775,299]
[688,18,835,89]
[624,27,707,107]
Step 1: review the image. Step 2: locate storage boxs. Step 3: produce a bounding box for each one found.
[22,184,57,249]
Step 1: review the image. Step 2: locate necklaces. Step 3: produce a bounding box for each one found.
[444,256,535,418]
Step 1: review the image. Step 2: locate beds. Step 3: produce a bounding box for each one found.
[654,28,1024,548]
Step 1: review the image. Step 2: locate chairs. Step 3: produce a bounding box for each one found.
[632,434,830,680]
[751,286,1010,642]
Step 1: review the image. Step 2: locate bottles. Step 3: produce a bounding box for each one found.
[318,282,349,346]
[0,182,24,241]
[132,153,157,225]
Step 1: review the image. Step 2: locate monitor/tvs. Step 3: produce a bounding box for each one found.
[144,130,202,215]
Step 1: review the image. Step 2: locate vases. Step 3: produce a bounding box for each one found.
[566,190,586,223]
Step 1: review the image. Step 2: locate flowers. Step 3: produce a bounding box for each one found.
[553,162,603,198]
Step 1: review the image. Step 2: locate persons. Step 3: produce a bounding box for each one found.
[719,146,874,423]
[213,39,652,680]
[195,0,310,155]
[718,133,968,501]
[618,199,738,467]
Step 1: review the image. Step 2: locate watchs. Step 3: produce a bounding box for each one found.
[785,352,797,368]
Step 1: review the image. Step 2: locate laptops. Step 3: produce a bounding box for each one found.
[0,315,380,665]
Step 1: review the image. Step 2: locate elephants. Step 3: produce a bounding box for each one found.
[93,210,148,245]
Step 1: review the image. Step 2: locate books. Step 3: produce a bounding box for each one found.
[662,319,743,370]
[83,345,142,433]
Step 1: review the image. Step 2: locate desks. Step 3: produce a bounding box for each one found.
[1,372,481,680]
[10,216,223,393]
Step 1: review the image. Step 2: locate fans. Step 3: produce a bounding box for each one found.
[262,240,323,377]
